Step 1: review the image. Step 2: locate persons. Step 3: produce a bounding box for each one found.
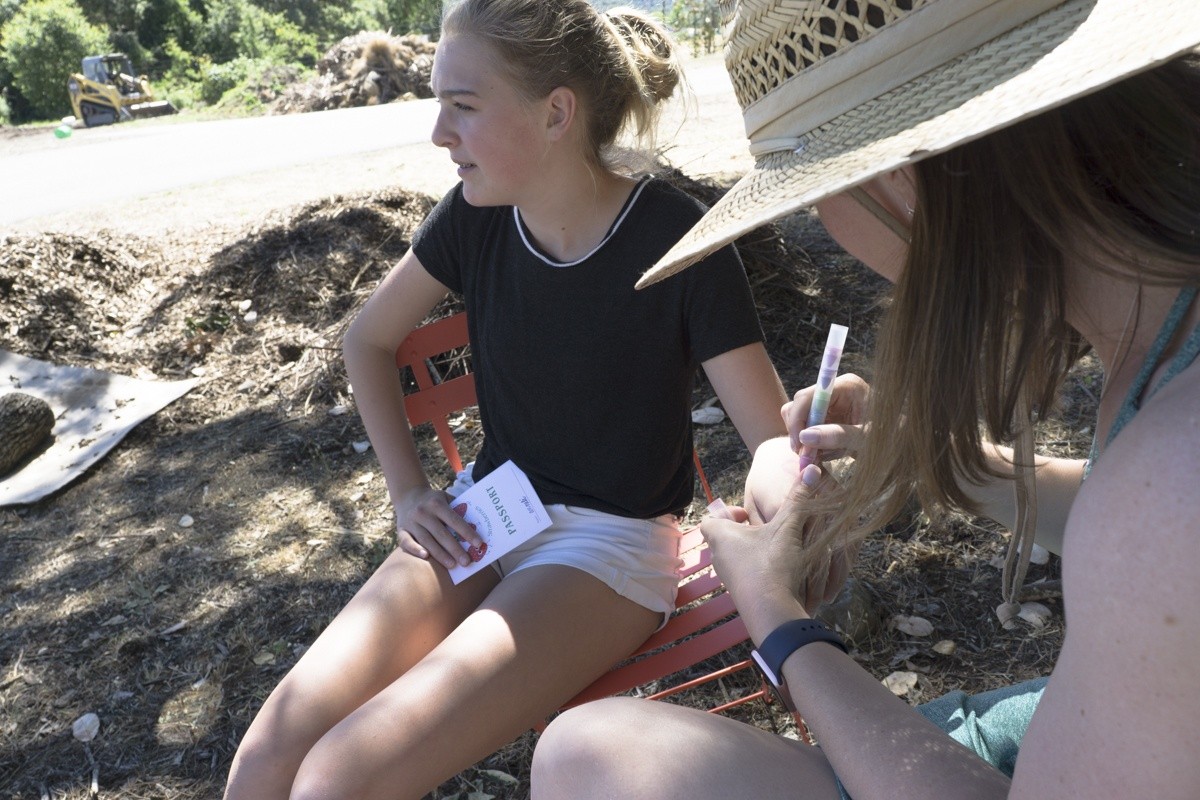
[529,0,1200,800]
[223,0,833,800]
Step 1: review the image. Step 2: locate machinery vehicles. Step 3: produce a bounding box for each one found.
[68,54,179,127]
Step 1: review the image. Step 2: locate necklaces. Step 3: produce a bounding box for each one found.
[1082,252,1154,485]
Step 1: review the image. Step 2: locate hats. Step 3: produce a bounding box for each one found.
[631,0,1200,290]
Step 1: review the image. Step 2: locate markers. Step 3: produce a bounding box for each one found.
[798,323,850,475]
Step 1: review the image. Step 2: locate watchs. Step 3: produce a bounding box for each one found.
[751,619,856,711]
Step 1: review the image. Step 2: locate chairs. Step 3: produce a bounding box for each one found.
[393,312,811,745]
